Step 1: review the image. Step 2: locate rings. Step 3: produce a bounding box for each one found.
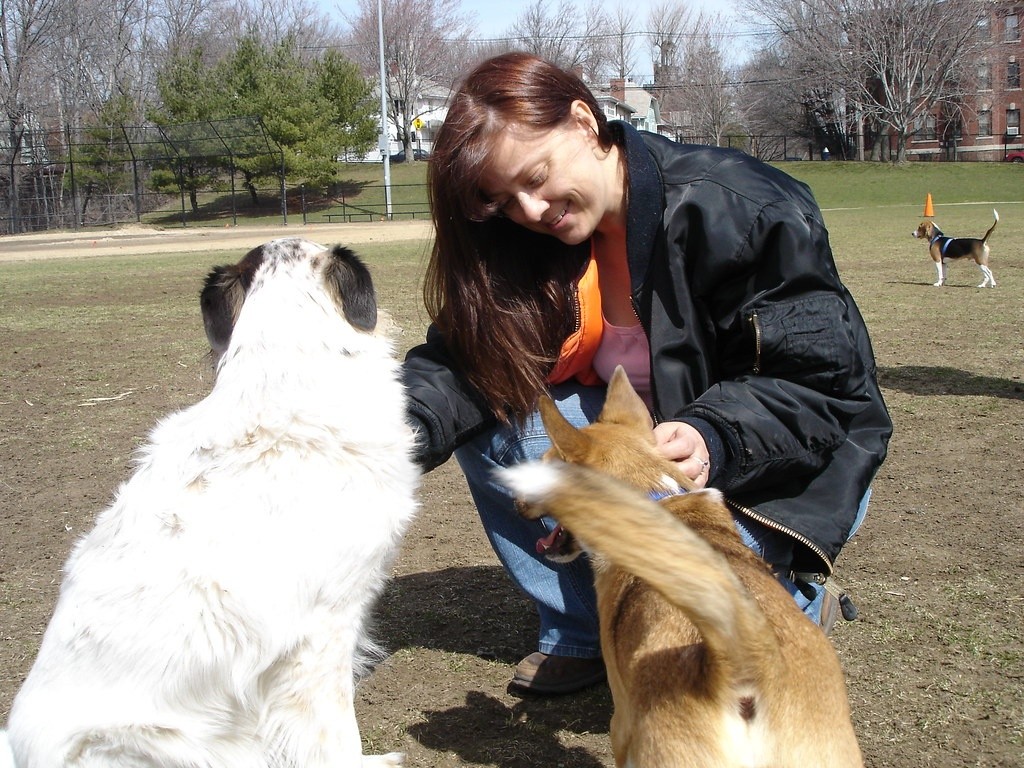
[692,456,708,471]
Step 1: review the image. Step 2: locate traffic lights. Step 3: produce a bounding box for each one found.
[416,119,421,130]
[411,131,415,142]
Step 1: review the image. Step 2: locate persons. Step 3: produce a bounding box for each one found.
[395,49,893,699]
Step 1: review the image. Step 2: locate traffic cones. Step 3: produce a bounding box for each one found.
[922,193,935,217]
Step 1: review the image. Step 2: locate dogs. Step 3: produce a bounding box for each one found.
[485,364,868,768]
[0,238,426,768]
[912,208,999,289]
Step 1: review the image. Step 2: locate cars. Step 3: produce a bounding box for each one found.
[390,148,430,161]
[1002,149,1024,164]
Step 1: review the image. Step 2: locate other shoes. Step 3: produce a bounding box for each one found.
[513,651,609,692]
[819,577,838,636]
